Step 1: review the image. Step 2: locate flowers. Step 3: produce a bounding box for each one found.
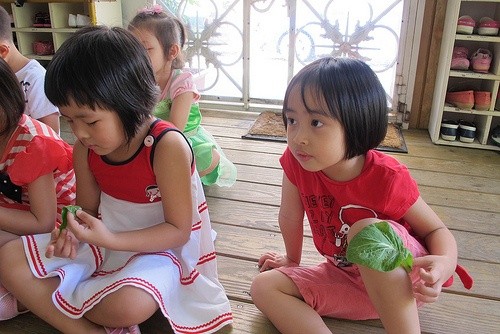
[137,4,163,16]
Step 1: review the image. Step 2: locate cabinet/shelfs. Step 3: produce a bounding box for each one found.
[428,0,500,151]
[2,0,122,66]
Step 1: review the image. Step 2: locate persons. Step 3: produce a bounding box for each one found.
[250,57,458,334]
[128,8,237,187]
[0,26,235,334]
[0,57,77,321]
[0,5,61,138]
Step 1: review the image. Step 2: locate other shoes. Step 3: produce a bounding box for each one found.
[0,286,30,321]
[441,15,500,146]
[104,321,141,334]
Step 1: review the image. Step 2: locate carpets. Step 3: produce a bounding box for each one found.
[241,106,410,155]
